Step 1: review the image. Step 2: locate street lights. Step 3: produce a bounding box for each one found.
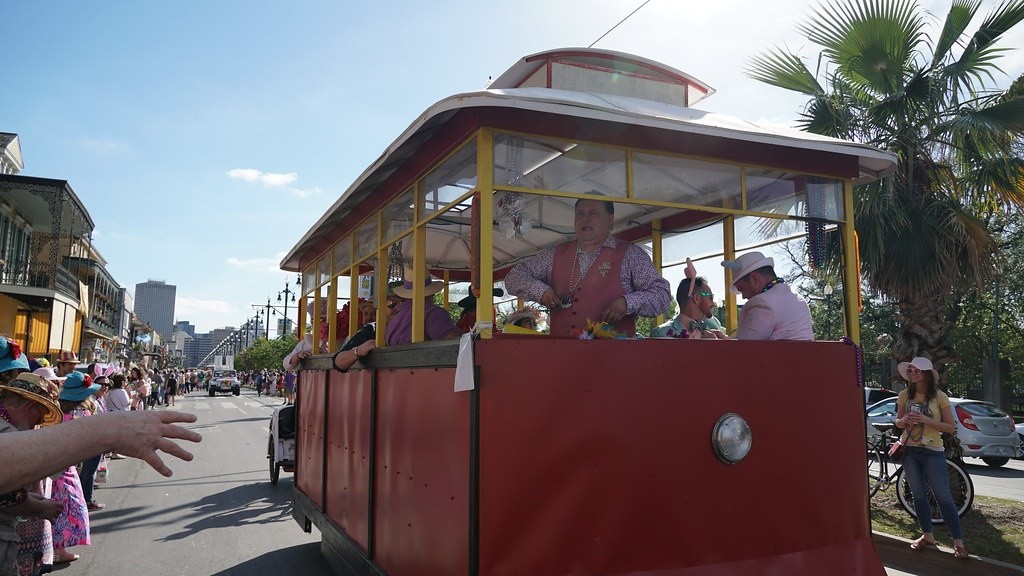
[197,315,253,368]
[276,275,295,348]
[261,293,276,342]
[250,307,263,340]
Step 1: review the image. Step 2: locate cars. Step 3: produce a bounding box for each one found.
[866,395,1020,467]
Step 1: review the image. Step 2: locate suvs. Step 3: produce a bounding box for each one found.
[209,369,241,397]
[864,386,899,408]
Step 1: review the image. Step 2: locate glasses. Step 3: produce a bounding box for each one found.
[387,299,405,308]
[98,382,110,387]
[907,368,922,375]
[699,294,714,303]
[35,404,46,425]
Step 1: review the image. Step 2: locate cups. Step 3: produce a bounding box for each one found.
[911,405,920,424]
[304,333,314,350]
[130,391,136,396]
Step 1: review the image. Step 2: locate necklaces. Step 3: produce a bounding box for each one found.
[841,336,862,386]
[504,136,522,226]
[763,277,784,292]
[805,177,826,270]
[0,405,12,423]
[560,241,602,309]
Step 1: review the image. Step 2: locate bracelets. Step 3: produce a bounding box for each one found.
[297,351,302,359]
[355,347,360,358]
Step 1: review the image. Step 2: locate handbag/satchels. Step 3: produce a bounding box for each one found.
[96,455,108,484]
[887,441,905,463]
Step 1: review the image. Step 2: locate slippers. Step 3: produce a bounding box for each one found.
[955,546,969,558]
[911,537,939,550]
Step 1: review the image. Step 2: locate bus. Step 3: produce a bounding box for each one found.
[267,47,903,576]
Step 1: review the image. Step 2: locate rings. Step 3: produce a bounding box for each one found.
[551,302,554,305]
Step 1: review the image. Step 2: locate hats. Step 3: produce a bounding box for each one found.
[360,296,374,311]
[898,357,938,382]
[0,337,102,425]
[393,264,444,298]
[458,284,504,308]
[721,252,774,294]
[505,306,547,332]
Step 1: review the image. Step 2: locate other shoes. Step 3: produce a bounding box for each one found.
[283,401,293,405]
[53,550,79,562]
[111,454,126,459]
[91,504,106,510]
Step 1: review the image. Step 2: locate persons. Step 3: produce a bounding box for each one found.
[702,249,813,340]
[238,368,297,405]
[896,357,968,557]
[505,190,673,336]
[283,295,376,370]
[29,356,212,564]
[0,373,64,576]
[0,411,202,493]
[650,257,726,338]
[332,281,406,373]
[0,338,53,576]
[456,285,504,333]
[385,266,457,345]
[506,306,540,329]
